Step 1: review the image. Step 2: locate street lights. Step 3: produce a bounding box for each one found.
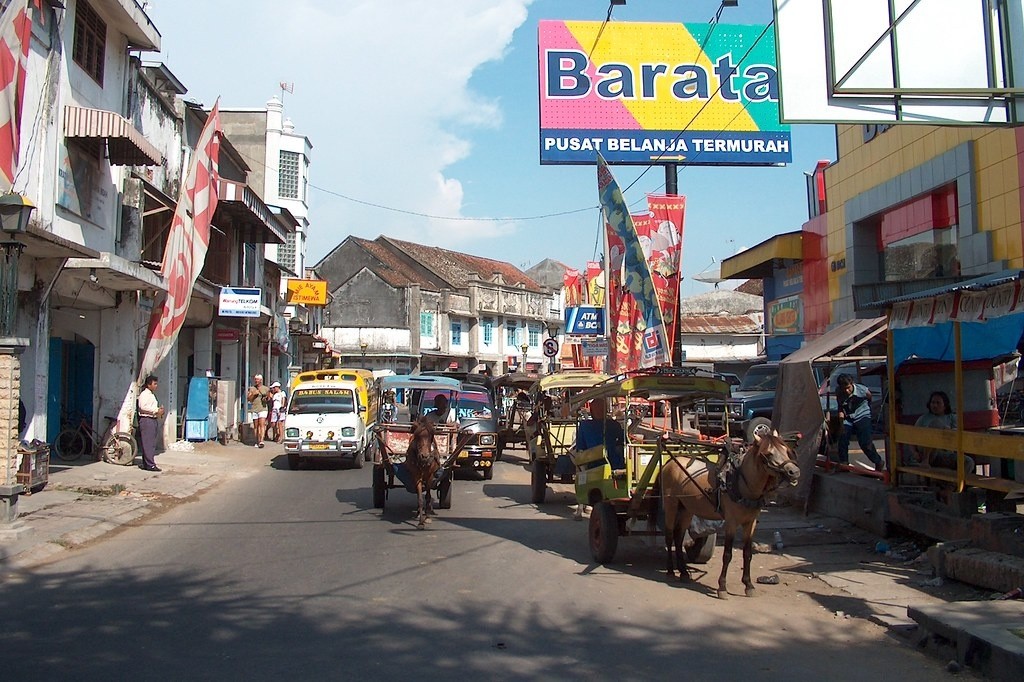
[545,323,561,373]
[360,343,368,369]
[520,343,529,373]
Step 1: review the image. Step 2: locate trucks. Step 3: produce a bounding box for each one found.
[648,362,741,417]
[408,371,506,480]
[283,368,378,470]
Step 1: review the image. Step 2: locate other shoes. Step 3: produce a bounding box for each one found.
[254,443,258,448]
[277,438,282,443]
[875,460,885,472]
[264,437,272,441]
[259,442,264,448]
[143,464,149,469]
[148,465,161,471]
[274,435,279,441]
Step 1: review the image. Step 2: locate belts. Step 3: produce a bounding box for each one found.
[141,416,157,419]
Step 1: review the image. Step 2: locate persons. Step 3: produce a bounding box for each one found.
[246,375,287,449]
[578,399,625,470]
[908,392,974,499]
[137,376,165,471]
[384,386,463,434]
[829,373,885,473]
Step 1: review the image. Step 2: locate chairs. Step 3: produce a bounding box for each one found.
[176,407,186,441]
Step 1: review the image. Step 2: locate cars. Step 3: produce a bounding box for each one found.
[818,360,886,434]
[687,362,843,445]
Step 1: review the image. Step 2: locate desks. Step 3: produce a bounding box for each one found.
[987,426,1024,513]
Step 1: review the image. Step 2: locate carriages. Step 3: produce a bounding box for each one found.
[518,372,644,522]
[570,366,800,600]
[371,375,474,530]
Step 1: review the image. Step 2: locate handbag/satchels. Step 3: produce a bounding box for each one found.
[279,390,288,412]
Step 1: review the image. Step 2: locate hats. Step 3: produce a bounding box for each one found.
[433,394,449,407]
[270,381,281,388]
[590,398,607,420]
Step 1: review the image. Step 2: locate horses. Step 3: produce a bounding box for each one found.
[404,416,443,531]
[648,430,800,600]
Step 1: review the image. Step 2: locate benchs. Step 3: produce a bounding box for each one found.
[526,412,627,490]
[893,409,1024,500]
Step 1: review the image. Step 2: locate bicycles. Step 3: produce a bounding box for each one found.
[53,414,137,465]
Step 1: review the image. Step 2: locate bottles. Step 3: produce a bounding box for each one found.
[774,531,783,549]
